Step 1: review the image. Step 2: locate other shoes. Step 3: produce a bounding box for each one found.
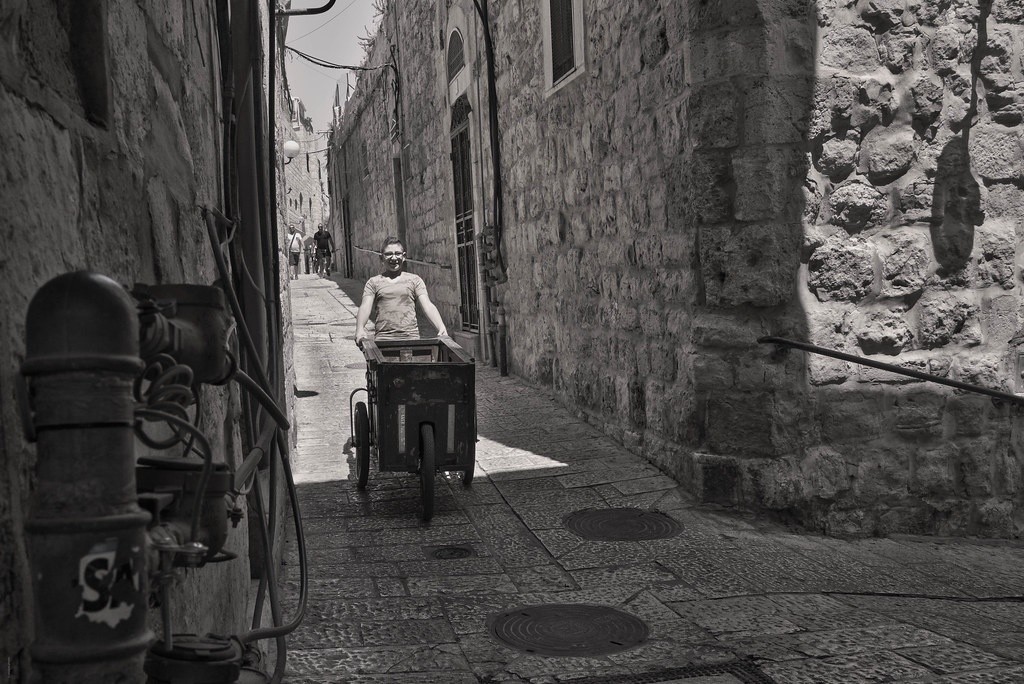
[325,269,330,276]
[295,275,298,280]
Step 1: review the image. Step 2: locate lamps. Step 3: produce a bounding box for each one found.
[297,216,304,224]
[284,141,300,164]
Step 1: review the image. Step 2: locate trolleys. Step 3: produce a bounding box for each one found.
[349,335,479,524]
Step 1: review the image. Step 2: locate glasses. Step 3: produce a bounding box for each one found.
[383,252,404,258]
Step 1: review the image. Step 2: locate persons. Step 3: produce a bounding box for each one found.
[355,236,448,346]
[286,223,336,279]
